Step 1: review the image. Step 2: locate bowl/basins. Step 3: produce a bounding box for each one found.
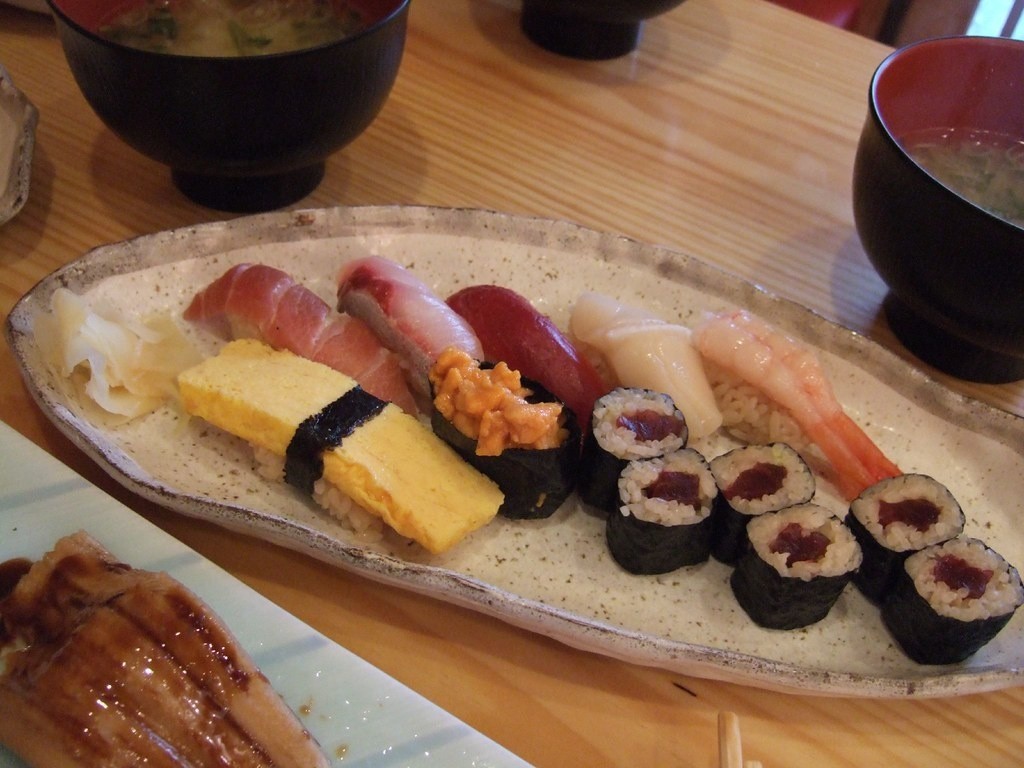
[519,0,686,59]
[48,0,408,210]
[849,36,1024,384]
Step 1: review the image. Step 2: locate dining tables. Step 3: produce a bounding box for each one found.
[0,0,1023,768]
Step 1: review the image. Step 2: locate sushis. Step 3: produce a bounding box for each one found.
[171,253,1024,666]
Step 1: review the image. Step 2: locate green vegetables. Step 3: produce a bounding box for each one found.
[100,6,363,54]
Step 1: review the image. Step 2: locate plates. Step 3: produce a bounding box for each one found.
[0,423,544,768]
[0,65,41,229]
[6,205,1024,699]
